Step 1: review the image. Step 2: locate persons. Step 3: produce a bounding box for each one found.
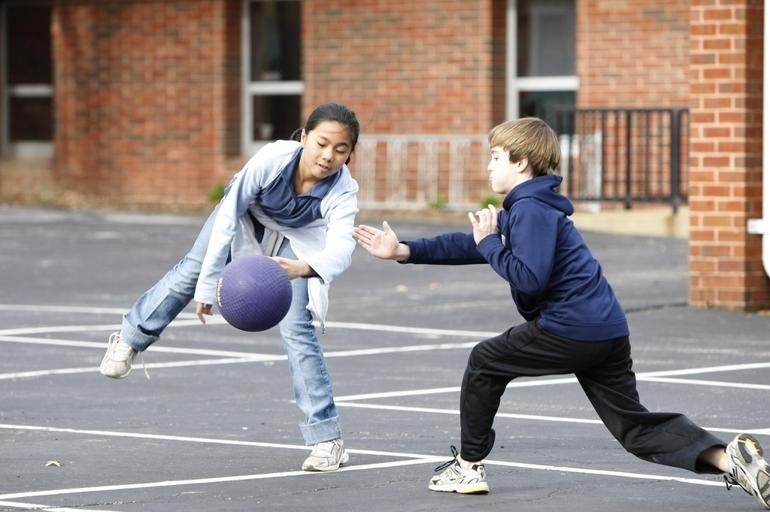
[352,117,770,510]
[99,103,359,472]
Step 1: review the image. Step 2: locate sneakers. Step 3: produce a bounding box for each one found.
[429,453,489,493]
[725,433,770,509]
[301,437,350,472]
[99,331,138,379]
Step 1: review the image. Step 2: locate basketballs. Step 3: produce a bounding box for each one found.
[216,254,293,333]
